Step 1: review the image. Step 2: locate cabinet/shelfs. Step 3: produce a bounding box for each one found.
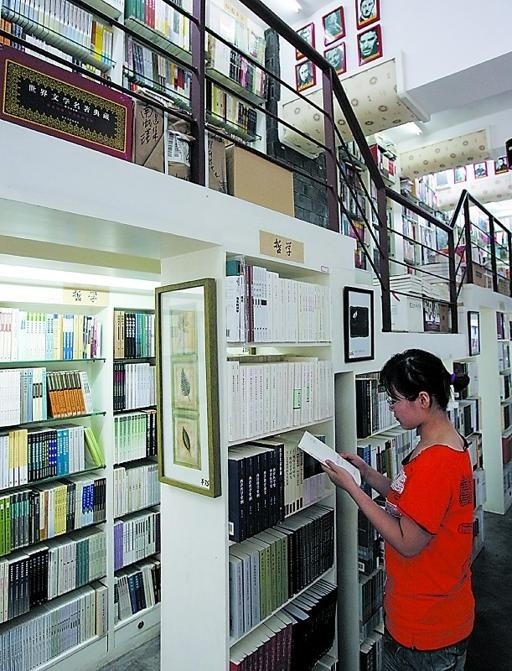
[479,309,512,517]
[333,355,485,671]
[336,132,401,277]
[0,0,267,152]
[470,215,512,282]
[163,253,336,670]
[0,260,162,670]
[402,177,448,275]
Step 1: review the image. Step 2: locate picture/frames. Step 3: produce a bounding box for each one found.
[452,167,467,185]
[321,6,347,47]
[294,59,317,94]
[322,42,348,77]
[294,21,316,61]
[354,0,381,31]
[356,23,383,67]
[152,276,227,500]
[493,156,509,176]
[341,287,377,364]
[466,311,481,357]
[473,161,488,181]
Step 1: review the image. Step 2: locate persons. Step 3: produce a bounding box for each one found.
[325,12,343,38]
[359,0,376,23]
[298,27,310,43]
[297,61,313,87]
[497,158,508,171]
[358,28,378,59]
[317,347,478,671]
[326,48,343,71]
[476,164,484,176]
[355,358,488,670]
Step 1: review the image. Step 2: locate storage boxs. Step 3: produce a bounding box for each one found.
[227,141,298,218]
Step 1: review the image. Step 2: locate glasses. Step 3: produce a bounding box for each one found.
[385,394,416,405]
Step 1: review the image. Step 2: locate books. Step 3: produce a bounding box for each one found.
[341,141,511,334]
[296,430,363,488]
[1,306,161,671]
[226,255,338,670]
[1,0,266,143]
[496,312,511,493]
